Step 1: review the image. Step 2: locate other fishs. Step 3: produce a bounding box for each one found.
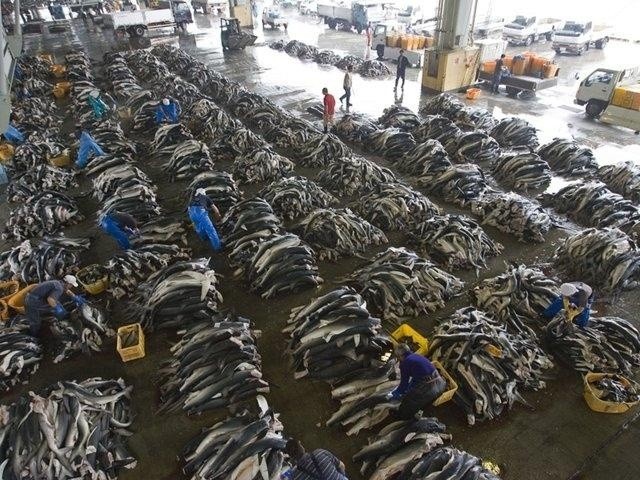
[0,38,640,480]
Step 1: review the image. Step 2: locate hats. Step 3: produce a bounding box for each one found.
[63,275,78,288]
[395,343,410,358]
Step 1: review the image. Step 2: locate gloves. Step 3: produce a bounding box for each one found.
[72,295,84,305]
[53,303,65,314]
[392,388,401,398]
[563,299,580,322]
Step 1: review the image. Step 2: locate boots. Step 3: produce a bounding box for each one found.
[333,125,337,132]
[322,127,327,133]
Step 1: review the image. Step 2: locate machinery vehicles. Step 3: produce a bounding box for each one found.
[220,17,258,50]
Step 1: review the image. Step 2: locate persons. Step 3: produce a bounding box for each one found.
[87,87,117,117]
[155,94,183,124]
[339,65,353,106]
[3,113,25,142]
[48,3,55,20]
[384,343,446,420]
[321,88,337,133]
[68,125,104,168]
[17,87,31,100]
[375,39,385,62]
[491,54,509,96]
[23,275,79,336]
[252,1,257,17]
[393,89,404,106]
[366,21,375,46]
[101,211,142,252]
[187,188,223,256]
[79,2,122,22]
[531,282,595,329]
[394,50,409,90]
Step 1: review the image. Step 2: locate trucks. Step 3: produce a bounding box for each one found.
[317,2,439,67]
[574,66,640,132]
[551,21,616,55]
[102,0,193,35]
[262,6,288,28]
[191,0,227,14]
[502,14,560,46]
[472,38,558,94]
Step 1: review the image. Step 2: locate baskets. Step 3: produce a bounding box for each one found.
[50,156,70,166]
[584,373,640,413]
[467,87,481,98]
[75,264,108,294]
[0,281,38,322]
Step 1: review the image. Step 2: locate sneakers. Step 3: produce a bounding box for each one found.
[340,98,352,106]
